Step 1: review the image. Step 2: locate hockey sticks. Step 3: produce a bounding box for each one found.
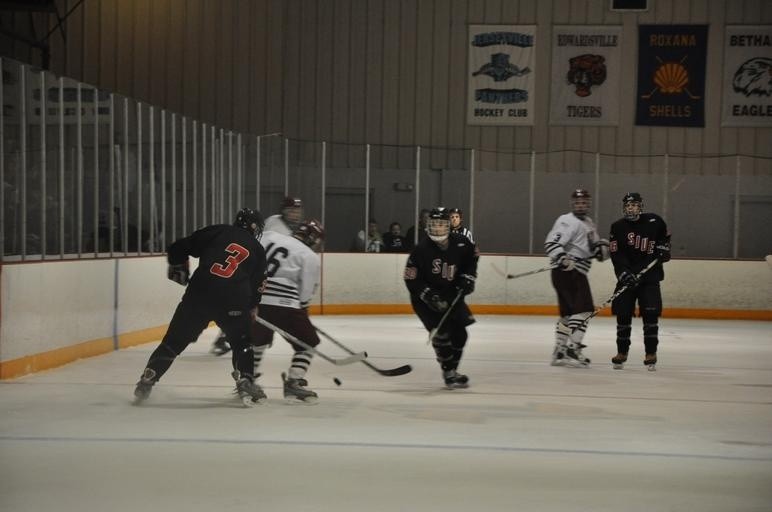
[255,316,368,364]
[425,287,463,346]
[313,324,412,377]
[491,253,595,278]
[558,257,658,334]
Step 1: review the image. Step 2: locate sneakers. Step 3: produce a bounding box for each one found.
[644,353,656,365]
[213,331,231,355]
[552,347,572,360]
[231,370,266,402]
[281,372,319,401]
[566,342,591,365]
[445,374,468,385]
[612,350,628,364]
[134,370,159,400]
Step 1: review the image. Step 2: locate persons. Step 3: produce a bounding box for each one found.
[380,222,409,254]
[209,198,304,357]
[405,208,478,391]
[611,192,671,371]
[133,207,268,407]
[357,217,385,253]
[545,189,606,368]
[251,218,327,406]
[3,188,156,256]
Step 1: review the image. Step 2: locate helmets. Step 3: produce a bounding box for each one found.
[569,187,592,217]
[424,207,451,241]
[278,195,304,230]
[449,208,464,229]
[622,192,646,220]
[290,217,326,255]
[233,208,265,243]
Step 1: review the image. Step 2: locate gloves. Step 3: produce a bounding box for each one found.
[655,241,671,259]
[419,287,450,312]
[594,239,610,262]
[168,261,190,286]
[616,267,641,289]
[452,273,477,295]
[557,255,576,271]
